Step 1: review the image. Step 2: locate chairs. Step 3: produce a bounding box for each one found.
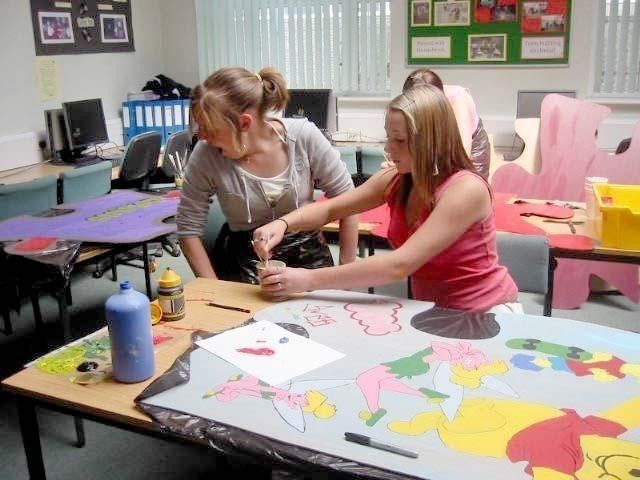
[0,174,59,336]
[361,145,388,294]
[200,192,227,253]
[331,146,358,186]
[93,130,162,278]
[407,233,554,317]
[155,129,194,256]
[57,160,119,306]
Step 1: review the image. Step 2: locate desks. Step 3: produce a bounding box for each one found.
[335,140,389,155]
[0,144,165,181]
[0,185,182,341]
[314,192,639,266]
[2,275,636,480]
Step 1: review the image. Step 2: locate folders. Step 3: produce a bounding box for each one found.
[183,99,191,132]
[174,100,183,132]
[144,101,154,132]
[135,101,145,136]
[153,101,165,145]
[122,101,135,145]
[163,100,174,145]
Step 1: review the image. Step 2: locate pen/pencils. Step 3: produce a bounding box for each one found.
[345,432,419,458]
[168,153,180,177]
[568,219,576,233]
[208,303,250,313]
[175,151,183,174]
[181,148,187,168]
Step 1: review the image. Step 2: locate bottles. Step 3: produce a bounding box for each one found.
[105,281,155,382]
[156,267,185,321]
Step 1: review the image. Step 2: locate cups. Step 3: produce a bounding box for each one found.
[584,176,607,220]
[256,261,286,297]
[175,174,184,189]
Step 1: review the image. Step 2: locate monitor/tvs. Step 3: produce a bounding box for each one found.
[62,97,109,162]
[282,87,333,132]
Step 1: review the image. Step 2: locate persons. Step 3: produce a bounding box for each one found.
[382,69,489,182]
[174,68,357,291]
[253,83,525,314]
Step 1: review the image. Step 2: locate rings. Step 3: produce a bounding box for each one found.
[258,237,267,241]
[253,239,260,243]
[275,282,283,291]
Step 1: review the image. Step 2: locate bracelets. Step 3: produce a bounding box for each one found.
[277,218,288,232]
